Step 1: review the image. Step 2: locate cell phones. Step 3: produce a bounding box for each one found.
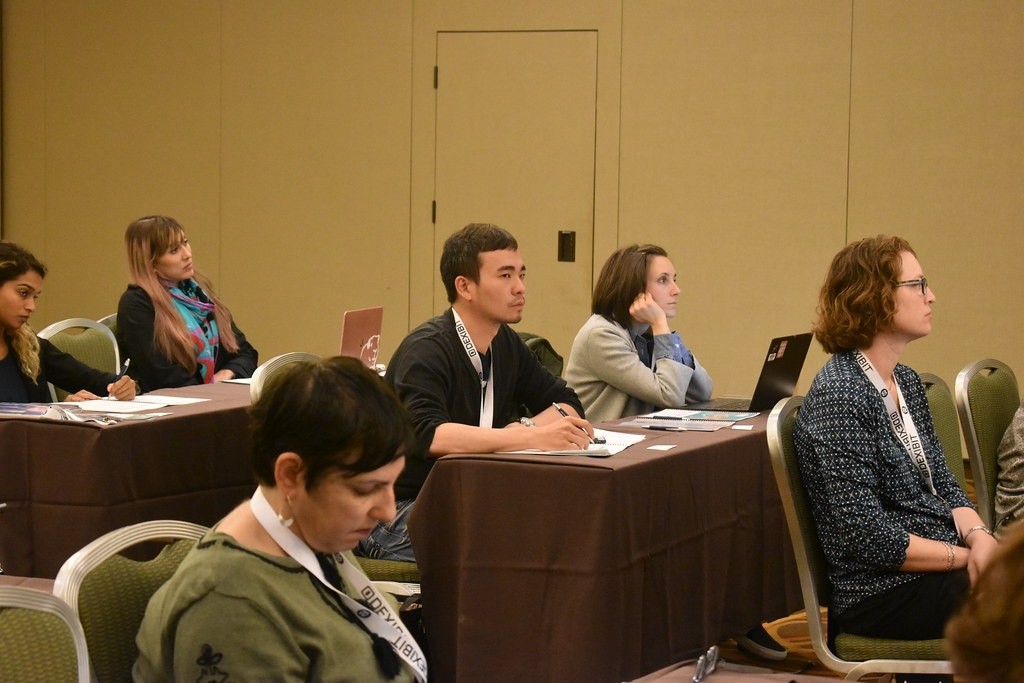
[590,435,606,444]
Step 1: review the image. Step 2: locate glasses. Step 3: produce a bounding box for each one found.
[692,646,718,682]
[892,278,929,294]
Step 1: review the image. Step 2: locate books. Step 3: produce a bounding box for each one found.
[50,395,210,412]
[616,408,760,431]
[493,428,646,455]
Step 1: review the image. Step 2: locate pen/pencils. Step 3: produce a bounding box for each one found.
[107,358,131,400]
[641,426,687,431]
[552,402,595,444]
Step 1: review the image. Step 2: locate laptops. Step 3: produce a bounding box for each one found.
[681,331,813,412]
[339,306,383,367]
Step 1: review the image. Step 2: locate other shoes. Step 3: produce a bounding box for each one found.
[732,624,787,661]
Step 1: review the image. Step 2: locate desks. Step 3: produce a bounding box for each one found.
[0,377,260,580]
[408,408,804,683]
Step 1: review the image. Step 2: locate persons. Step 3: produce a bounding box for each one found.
[0,242,135,403]
[566,244,714,424]
[117,215,259,391]
[358,223,595,563]
[795,235,999,639]
[944,523,1024,683]
[132,355,415,683]
[995,399,1024,542]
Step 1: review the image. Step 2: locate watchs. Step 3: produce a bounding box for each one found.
[516,417,536,428]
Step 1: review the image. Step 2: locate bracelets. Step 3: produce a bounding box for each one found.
[963,525,993,544]
[940,541,955,572]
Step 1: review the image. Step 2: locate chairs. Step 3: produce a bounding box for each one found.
[354,555,421,620]
[97,311,117,338]
[765,393,955,683]
[919,372,967,497]
[0,584,91,683]
[53,518,213,683]
[36,318,121,403]
[954,358,1021,534]
